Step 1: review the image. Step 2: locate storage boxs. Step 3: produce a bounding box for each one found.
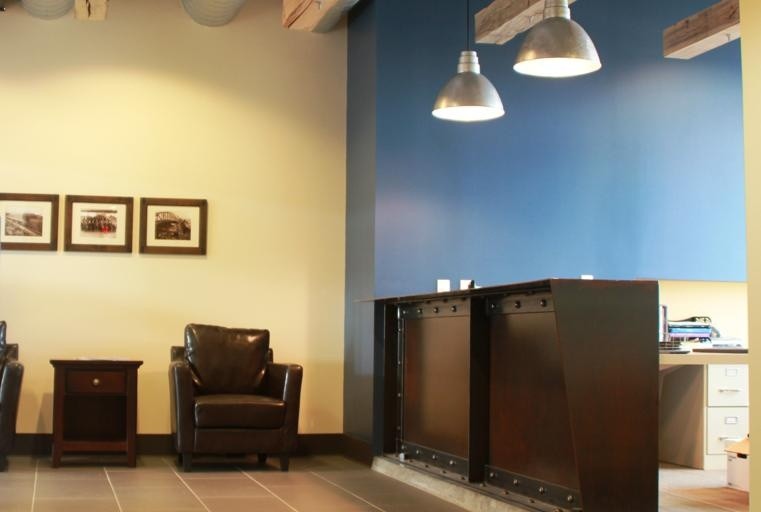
[726,452,749,492]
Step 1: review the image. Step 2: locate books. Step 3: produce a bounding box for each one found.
[659,316,749,355]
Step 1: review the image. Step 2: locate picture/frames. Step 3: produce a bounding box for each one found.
[0,194,59,250]
[65,195,132,252]
[139,198,207,254]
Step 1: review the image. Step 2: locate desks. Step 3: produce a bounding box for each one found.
[352,279,659,512]
[659,353,749,470]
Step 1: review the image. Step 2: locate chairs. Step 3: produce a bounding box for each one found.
[0,344,23,472]
[171,347,302,471]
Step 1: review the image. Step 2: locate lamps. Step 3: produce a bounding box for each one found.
[432,0,505,122]
[513,0,602,78]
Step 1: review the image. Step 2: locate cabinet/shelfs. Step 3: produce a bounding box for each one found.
[50,361,143,467]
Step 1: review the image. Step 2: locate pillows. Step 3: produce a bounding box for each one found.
[185,324,269,396]
[0,321,6,385]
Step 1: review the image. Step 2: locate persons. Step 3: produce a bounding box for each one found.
[81,215,116,233]
